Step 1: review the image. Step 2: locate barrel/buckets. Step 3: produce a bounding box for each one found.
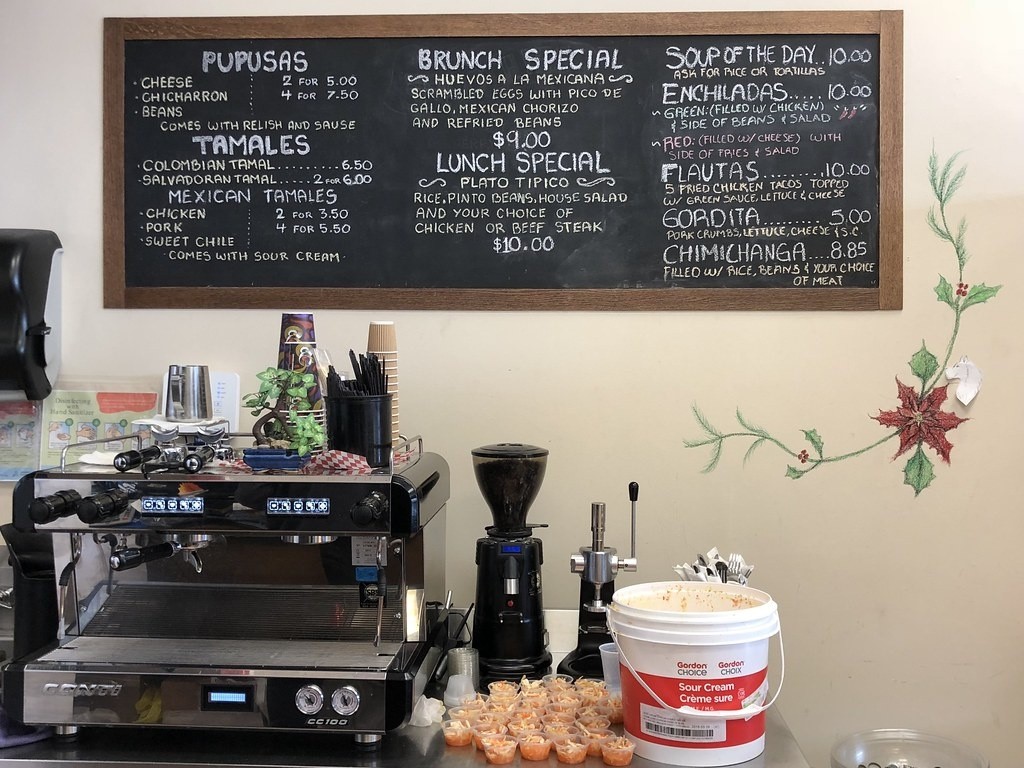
[605,580,785,767]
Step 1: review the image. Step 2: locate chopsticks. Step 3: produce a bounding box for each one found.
[325,348,389,397]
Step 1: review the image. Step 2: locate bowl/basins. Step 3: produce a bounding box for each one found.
[443,674,636,767]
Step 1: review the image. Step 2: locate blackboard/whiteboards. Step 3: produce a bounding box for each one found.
[101,9,904,314]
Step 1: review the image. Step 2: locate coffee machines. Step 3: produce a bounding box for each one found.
[471,446,554,694]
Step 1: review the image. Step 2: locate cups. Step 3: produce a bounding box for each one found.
[323,394,393,467]
[366,320,400,456]
[165,364,213,423]
[830,727,990,768]
[276,311,328,457]
[599,643,621,690]
